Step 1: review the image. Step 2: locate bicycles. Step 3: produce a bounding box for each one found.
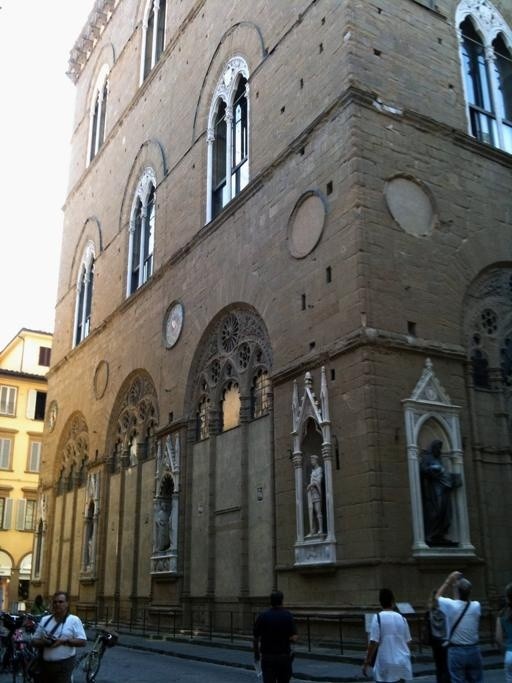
[0,609,52,683]
[68,622,119,682]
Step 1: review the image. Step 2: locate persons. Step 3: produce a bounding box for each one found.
[9,591,87,683]
[253,588,300,683]
[362,587,413,683]
[420,437,462,547]
[427,571,512,683]
[305,454,323,537]
